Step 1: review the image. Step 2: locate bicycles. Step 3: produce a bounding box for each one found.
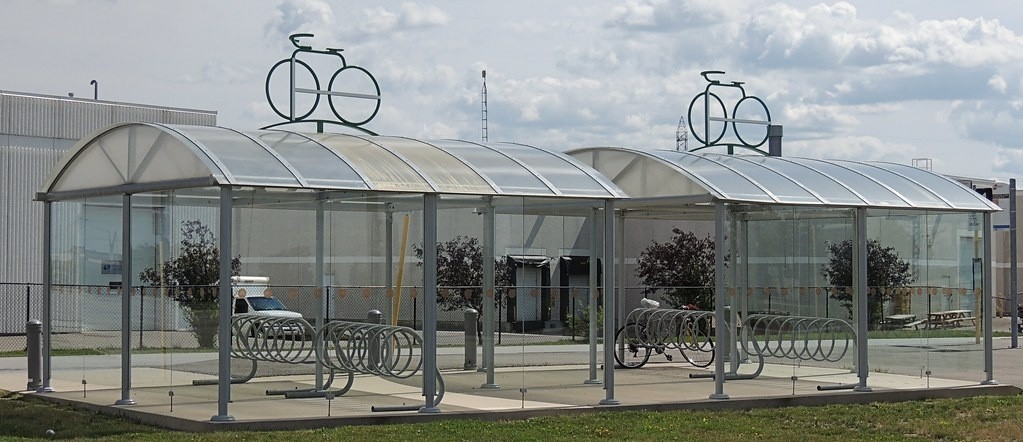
[613,296,715,369]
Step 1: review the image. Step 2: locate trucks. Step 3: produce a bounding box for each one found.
[212,275,305,341]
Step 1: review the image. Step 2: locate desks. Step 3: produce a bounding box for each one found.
[927,310,976,331]
[884,314,919,331]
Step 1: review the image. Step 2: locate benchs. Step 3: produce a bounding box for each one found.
[924,316,975,322]
[903,321,921,328]
[880,321,893,323]
